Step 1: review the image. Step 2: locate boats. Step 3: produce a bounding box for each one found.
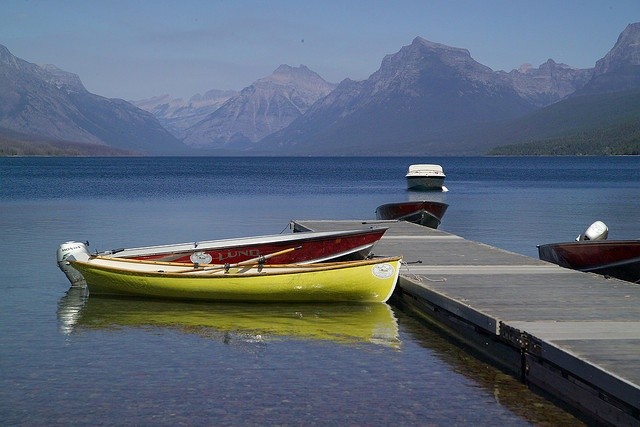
[406,164,446,188]
[536,240,640,282]
[56,227,388,289]
[375,200,450,230]
[66,256,402,302]
[74,301,402,351]
